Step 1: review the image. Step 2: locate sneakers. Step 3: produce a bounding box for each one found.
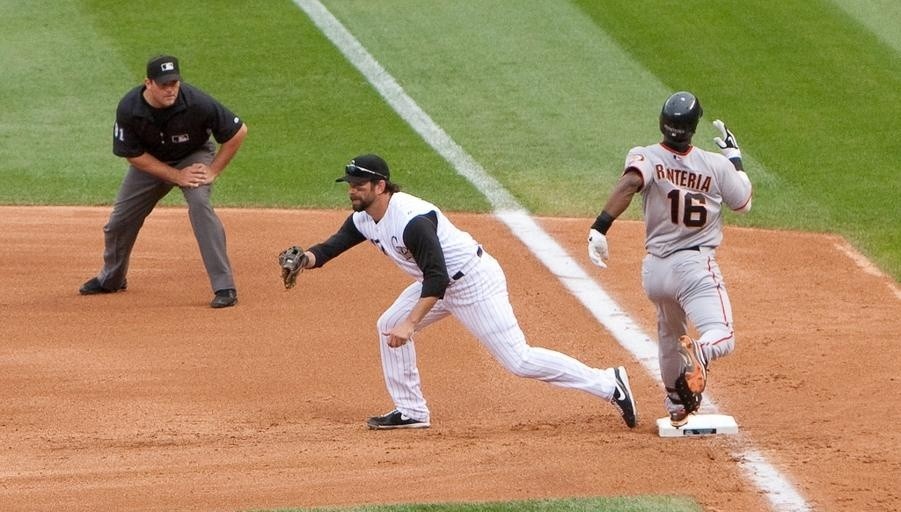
[367,409,432,429]
[610,365,636,429]
[675,333,709,395]
[664,393,703,427]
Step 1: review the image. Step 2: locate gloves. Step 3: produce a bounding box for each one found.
[586,228,610,269]
[712,118,742,160]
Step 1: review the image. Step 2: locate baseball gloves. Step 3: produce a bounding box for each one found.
[279,245,306,289]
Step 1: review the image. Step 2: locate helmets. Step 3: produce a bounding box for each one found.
[658,89,704,145]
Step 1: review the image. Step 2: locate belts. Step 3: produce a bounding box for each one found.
[677,245,700,251]
[447,246,484,287]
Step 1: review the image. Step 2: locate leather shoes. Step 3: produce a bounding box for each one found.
[210,288,238,308]
[78,276,128,295]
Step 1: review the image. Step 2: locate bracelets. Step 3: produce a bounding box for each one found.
[590,209,612,236]
[727,158,742,171]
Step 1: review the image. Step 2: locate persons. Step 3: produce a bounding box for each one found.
[278,154,638,430]
[587,92,751,425]
[77,54,248,309]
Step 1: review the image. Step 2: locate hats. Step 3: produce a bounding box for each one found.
[145,54,184,87]
[335,154,391,184]
